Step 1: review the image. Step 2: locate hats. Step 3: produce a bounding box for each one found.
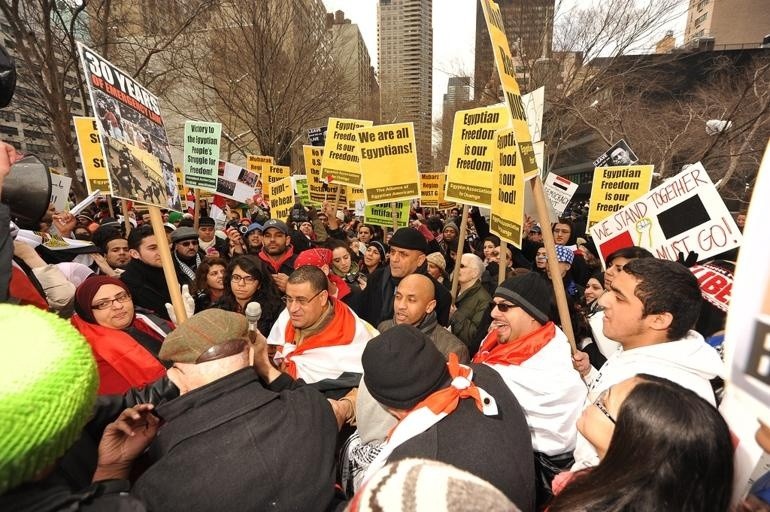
[538,245,575,266]
[426,251,450,280]
[441,221,459,237]
[157,308,249,364]
[199,218,215,227]
[388,227,430,255]
[367,240,389,261]
[263,218,289,238]
[169,227,200,243]
[492,271,552,327]
[362,323,449,409]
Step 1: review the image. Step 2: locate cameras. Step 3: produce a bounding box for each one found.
[291,203,309,221]
[345,271,360,284]
[226,218,249,236]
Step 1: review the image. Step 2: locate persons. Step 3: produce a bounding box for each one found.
[0,135,769,512]
[93,89,144,151]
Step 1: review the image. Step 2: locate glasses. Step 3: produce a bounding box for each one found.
[552,228,571,236]
[230,274,259,284]
[176,241,199,246]
[91,291,133,310]
[459,264,478,274]
[488,301,519,313]
[281,289,326,306]
[490,250,509,260]
[591,389,616,427]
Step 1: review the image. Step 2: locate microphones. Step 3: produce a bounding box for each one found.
[245,302,262,366]
